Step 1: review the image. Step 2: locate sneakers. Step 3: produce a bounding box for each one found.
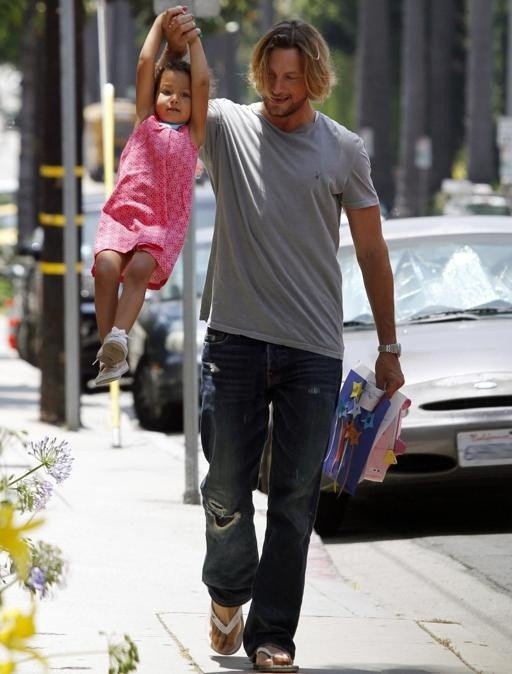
[96,330,130,368]
[94,358,131,386]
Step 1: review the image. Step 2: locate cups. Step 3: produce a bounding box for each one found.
[362,381,385,411]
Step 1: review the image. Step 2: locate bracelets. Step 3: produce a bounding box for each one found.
[378,344,401,356]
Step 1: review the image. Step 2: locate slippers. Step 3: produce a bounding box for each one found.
[251,640,300,673]
[206,594,248,656]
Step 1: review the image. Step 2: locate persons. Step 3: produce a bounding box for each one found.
[95,7,210,385]
[160,6,405,672]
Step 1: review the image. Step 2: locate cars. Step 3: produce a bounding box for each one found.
[436,174,512,219]
[258,204,512,552]
